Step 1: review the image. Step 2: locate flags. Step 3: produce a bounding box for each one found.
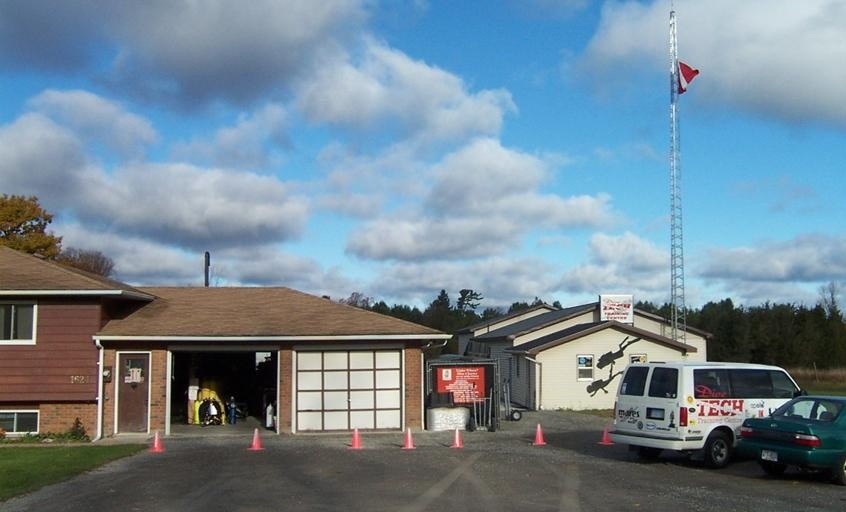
[676,59,699,94]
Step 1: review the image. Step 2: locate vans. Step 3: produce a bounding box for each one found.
[611,361,808,469]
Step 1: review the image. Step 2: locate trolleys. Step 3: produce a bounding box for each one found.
[501,378,521,421]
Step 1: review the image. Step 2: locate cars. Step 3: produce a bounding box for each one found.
[741,396,846,484]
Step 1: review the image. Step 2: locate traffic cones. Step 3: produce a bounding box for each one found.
[450,428,463,449]
[348,428,364,449]
[247,428,265,451]
[400,427,416,450]
[597,422,615,446]
[149,433,165,453]
[532,423,547,445]
